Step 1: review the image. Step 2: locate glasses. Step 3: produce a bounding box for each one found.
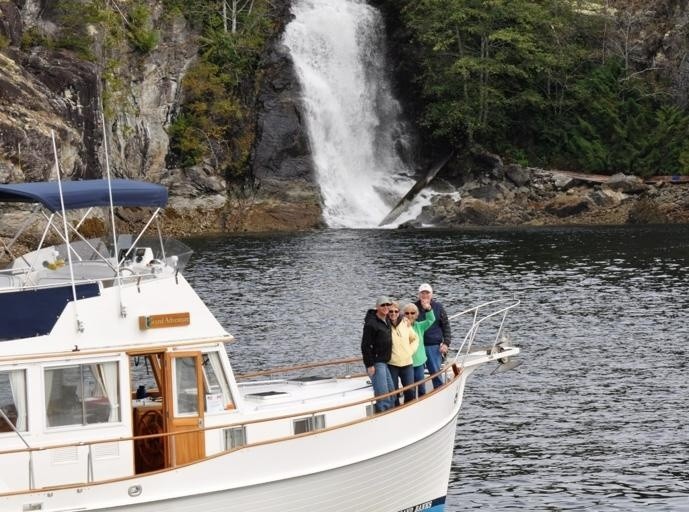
[389,310,399,313]
[405,312,415,315]
[380,303,390,307]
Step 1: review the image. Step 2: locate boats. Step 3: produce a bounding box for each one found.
[0,178,522,512]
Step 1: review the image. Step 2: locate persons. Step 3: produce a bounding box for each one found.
[386,301,419,408]
[412,283,452,390]
[401,298,436,400]
[360,295,411,415]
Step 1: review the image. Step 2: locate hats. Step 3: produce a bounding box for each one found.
[419,284,432,293]
[377,297,390,304]
